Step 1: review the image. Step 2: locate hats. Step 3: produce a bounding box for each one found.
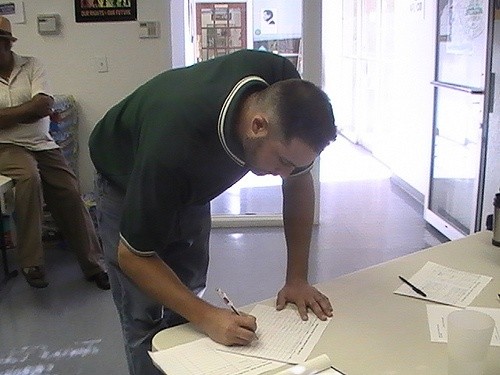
[0,17,17,42]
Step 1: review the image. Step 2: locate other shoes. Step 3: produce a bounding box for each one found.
[87,271,111,290]
[22,266,48,288]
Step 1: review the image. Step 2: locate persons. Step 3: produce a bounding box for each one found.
[87,49,337,375]
[0,16,111,291]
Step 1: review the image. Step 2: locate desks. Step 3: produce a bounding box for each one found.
[151,231,500,374]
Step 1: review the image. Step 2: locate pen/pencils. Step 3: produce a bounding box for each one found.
[217,288,259,341]
[399,276,426,296]
